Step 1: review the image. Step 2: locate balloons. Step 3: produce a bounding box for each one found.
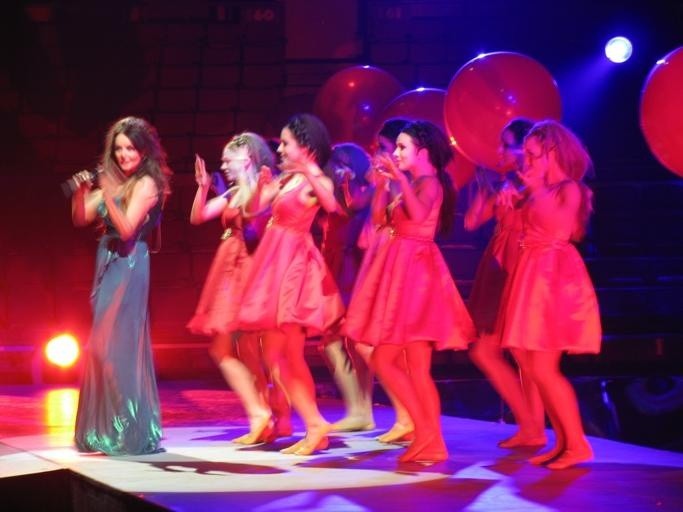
[377,86,474,190]
[441,50,565,174]
[640,45,683,178]
[315,62,405,156]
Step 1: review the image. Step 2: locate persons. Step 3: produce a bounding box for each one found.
[368,117,475,462]
[341,115,416,445]
[242,110,333,456]
[189,128,293,442]
[496,117,602,471]
[316,141,379,432]
[462,115,549,448]
[69,116,169,455]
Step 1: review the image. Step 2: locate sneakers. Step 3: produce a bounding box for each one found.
[232,412,449,461]
[498,432,593,469]
